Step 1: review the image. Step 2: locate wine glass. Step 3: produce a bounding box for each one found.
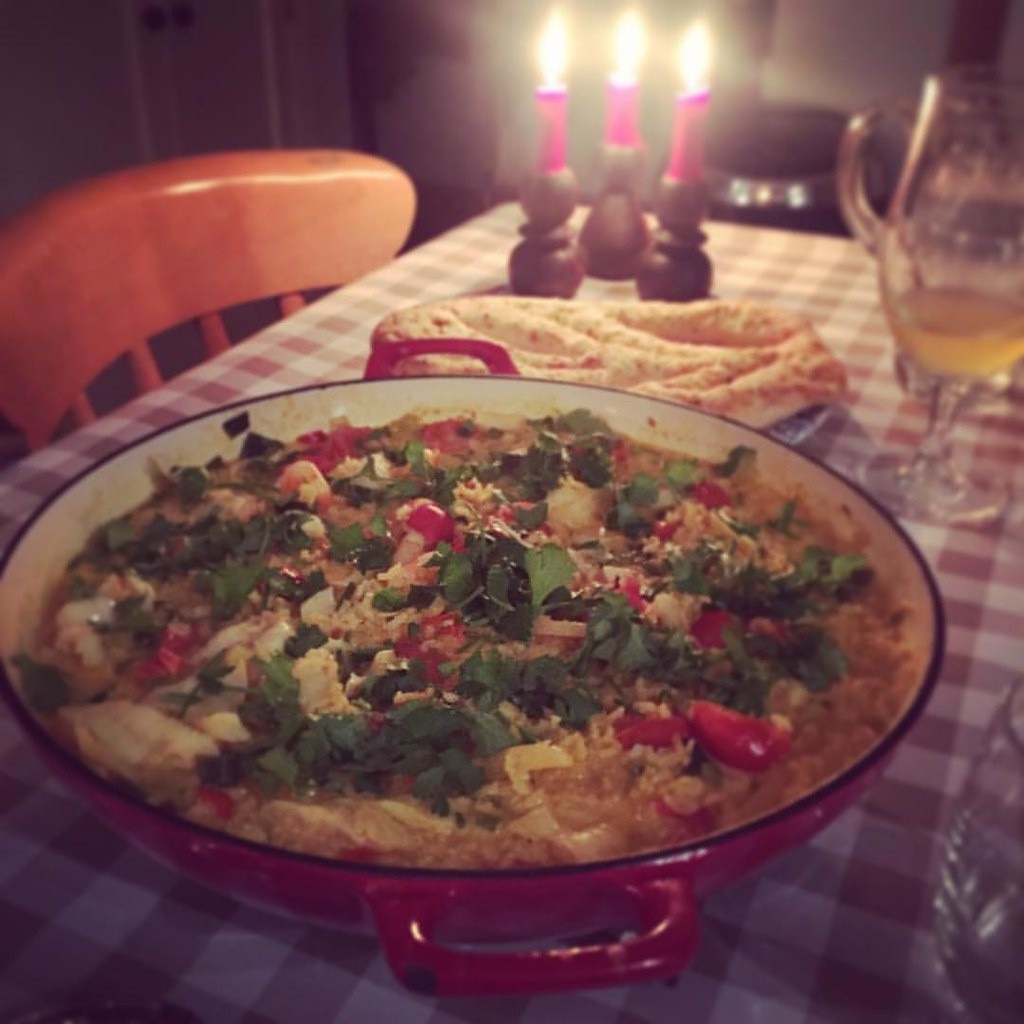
[842,62,1022,535]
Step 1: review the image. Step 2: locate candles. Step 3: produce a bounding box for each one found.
[669,20,715,183]
[534,6,577,170]
[606,6,646,144]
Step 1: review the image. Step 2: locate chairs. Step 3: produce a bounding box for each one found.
[0,149,420,462]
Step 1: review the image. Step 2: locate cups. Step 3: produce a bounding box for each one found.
[934,671,1024,1024]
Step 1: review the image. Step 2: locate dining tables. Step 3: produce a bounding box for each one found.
[0,199,1024,1024]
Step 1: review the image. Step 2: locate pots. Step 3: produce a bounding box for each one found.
[0,330,949,1005]
